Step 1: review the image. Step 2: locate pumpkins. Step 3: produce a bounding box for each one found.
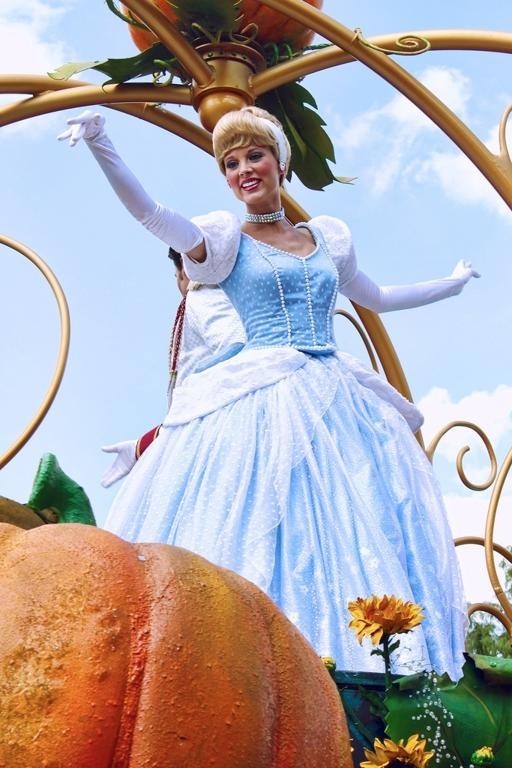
[124,0,324,53]
[0,522,355,768]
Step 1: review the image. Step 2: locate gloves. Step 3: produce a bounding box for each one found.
[56,110,106,148]
[99,438,139,488]
[451,259,481,293]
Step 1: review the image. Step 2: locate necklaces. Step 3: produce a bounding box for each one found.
[243,208,286,225]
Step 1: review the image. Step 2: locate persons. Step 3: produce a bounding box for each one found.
[55,104,482,684]
[99,247,190,489]
